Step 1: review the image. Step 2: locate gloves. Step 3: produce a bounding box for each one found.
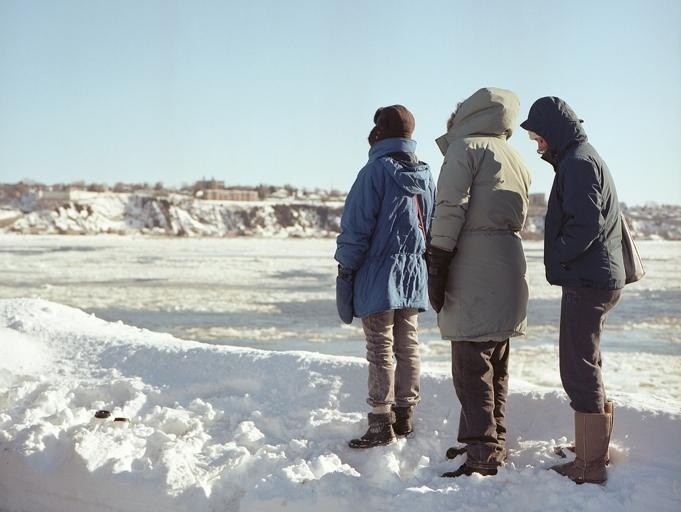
[427,247,458,314]
[336,264,354,324]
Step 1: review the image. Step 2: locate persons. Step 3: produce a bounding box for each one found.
[422,87,530,478]
[334,105,438,449]
[519,96,644,484]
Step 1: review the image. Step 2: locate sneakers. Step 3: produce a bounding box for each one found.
[446,448,467,459]
[443,464,498,477]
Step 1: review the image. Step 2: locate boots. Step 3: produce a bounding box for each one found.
[391,403,413,439]
[548,400,614,484]
[350,411,395,448]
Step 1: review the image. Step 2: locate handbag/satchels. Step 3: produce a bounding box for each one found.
[620,212,645,284]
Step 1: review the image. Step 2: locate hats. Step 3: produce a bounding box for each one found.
[367,105,415,145]
[528,130,540,140]
